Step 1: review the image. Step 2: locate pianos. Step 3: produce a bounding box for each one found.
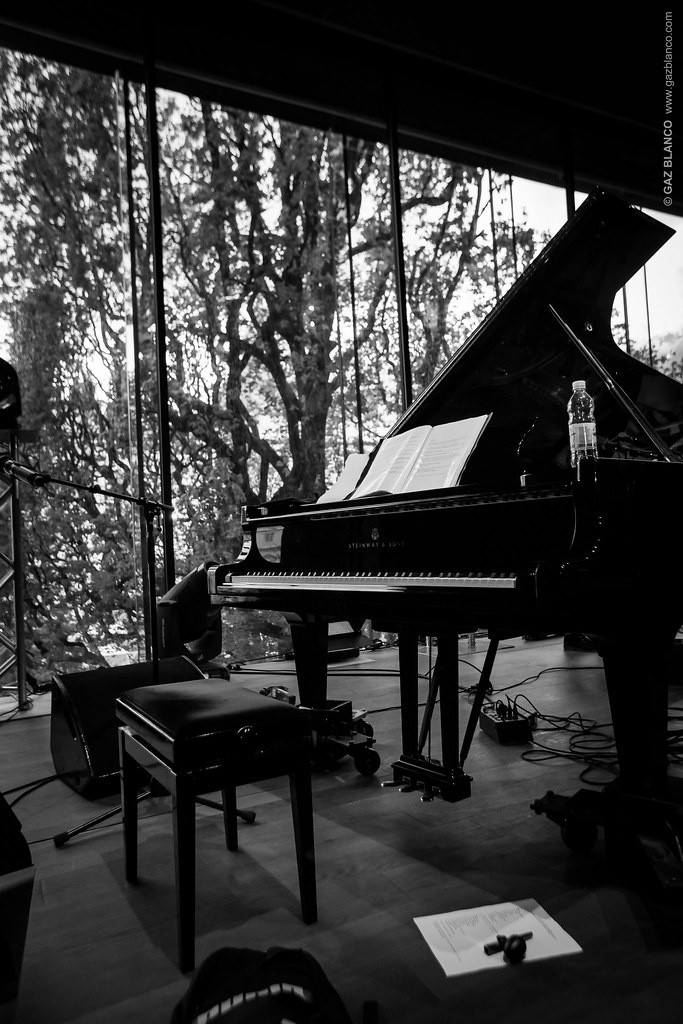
[200,186,683,839]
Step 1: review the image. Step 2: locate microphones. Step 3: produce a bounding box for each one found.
[0,452,43,488]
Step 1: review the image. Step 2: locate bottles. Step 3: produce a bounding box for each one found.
[567,379,597,467]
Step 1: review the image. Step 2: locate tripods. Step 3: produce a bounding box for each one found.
[53,476,257,846]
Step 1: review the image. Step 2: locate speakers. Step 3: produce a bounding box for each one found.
[50,653,205,800]
[328,621,356,663]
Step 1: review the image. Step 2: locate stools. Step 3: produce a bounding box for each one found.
[114,678,318,976]
